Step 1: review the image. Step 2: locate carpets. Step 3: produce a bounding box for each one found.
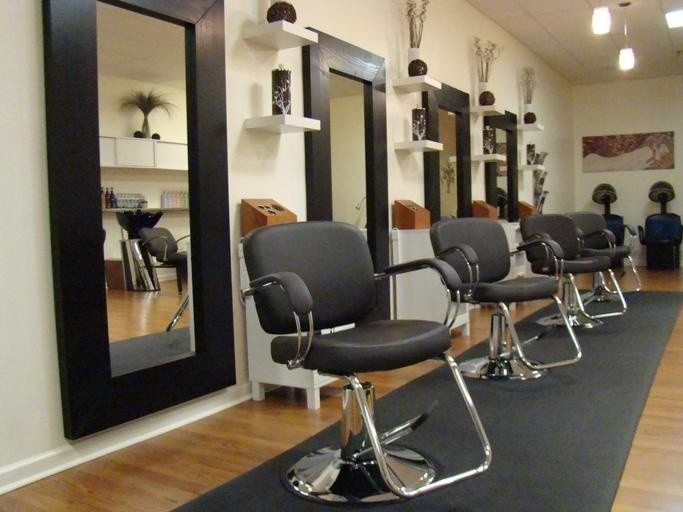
[163,288,683,512]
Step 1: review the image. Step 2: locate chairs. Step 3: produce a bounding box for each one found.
[566,212,646,305]
[425,215,586,382]
[516,212,632,331]
[241,218,502,505]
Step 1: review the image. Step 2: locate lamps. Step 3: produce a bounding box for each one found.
[591,0,635,71]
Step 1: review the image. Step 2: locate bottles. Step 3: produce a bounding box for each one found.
[98,186,187,209]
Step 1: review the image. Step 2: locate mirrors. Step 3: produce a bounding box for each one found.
[299,25,392,325]
[420,76,475,232]
[480,105,526,222]
[44,1,238,443]
